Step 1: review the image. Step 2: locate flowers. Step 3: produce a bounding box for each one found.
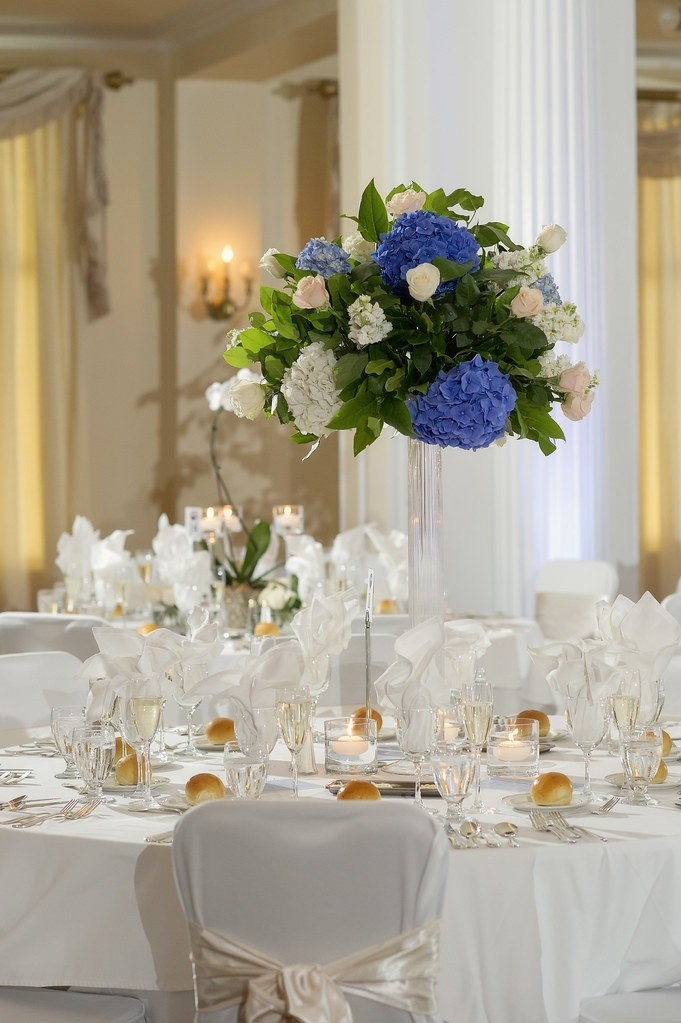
[191,535,307,607]
[201,184,599,449]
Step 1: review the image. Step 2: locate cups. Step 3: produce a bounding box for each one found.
[324,717,377,774]
[486,718,540,777]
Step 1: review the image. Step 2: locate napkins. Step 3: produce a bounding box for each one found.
[48,515,681,745]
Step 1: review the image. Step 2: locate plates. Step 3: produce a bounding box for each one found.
[491,728,569,741]
[501,792,591,810]
[604,772,681,790]
[157,794,190,809]
[331,727,396,739]
[149,752,179,769]
[194,738,236,751]
[611,747,681,762]
[102,775,170,792]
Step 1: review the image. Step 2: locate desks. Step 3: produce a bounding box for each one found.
[0,738,681,1023]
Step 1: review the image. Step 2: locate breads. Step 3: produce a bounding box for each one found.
[336,781,381,800]
[650,760,668,784]
[255,621,279,636]
[347,708,383,735]
[647,730,672,757]
[114,737,152,788]
[205,717,236,746]
[137,624,156,634]
[376,598,400,615]
[531,772,573,806]
[113,604,123,616]
[185,773,225,805]
[516,710,550,737]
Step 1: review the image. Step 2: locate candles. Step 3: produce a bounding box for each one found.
[322,720,383,778]
[200,506,219,531]
[277,506,301,527]
[224,510,240,530]
[486,717,542,780]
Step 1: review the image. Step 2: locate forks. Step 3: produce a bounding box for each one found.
[528,810,608,844]
[0,799,102,828]
[548,797,621,818]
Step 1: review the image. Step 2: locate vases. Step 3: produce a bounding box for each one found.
[404,435,445,742]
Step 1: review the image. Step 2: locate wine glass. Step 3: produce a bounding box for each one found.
[38,563,666,828]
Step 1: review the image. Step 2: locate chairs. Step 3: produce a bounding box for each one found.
[0,611,681,1023]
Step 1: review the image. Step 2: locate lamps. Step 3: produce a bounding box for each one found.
[197,247,253,319]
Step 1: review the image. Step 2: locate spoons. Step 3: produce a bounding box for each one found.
[0,794,28,812]
[458,821,480,848]
[493,822,520,847]
